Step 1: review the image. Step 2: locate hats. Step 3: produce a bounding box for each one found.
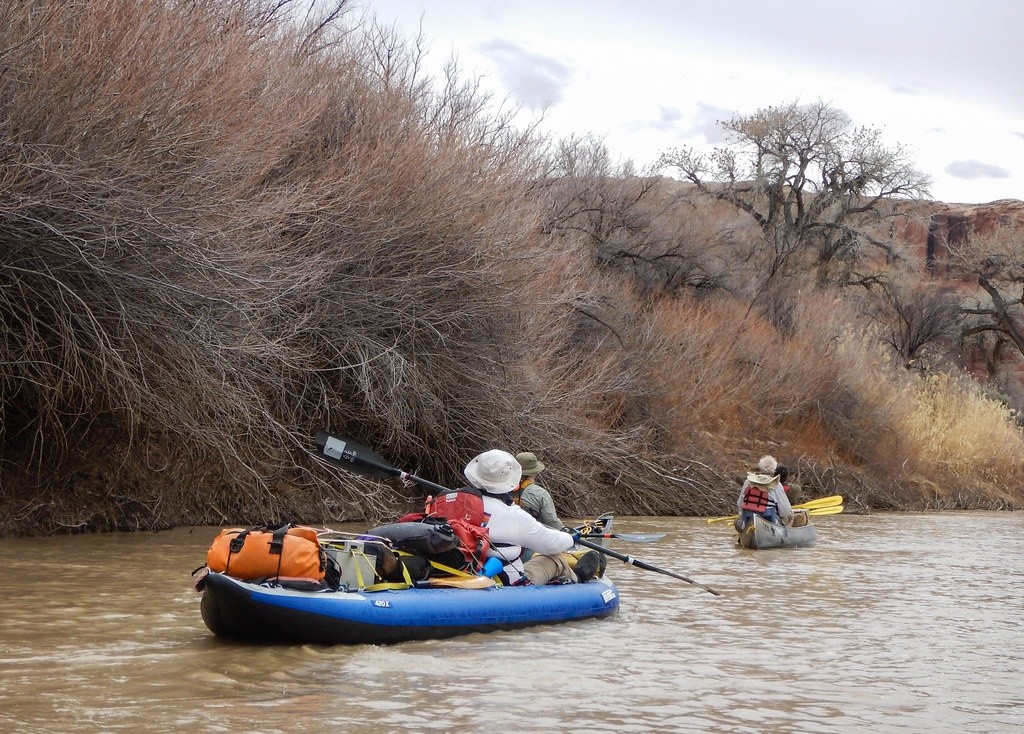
[514,451,544,475]
[746,471,781,491]
[463,448,523,494]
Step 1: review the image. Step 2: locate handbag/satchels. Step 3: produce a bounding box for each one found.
[206,521,326,580]
[396,488,490,570]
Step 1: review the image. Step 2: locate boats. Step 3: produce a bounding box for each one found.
[190,522,619,649]
[740,512,816,550]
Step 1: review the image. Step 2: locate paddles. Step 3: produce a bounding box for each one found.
[707,495,844,525]
[314,430,726,598]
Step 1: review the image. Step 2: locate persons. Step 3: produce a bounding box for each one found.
[462,448,601,586]
[734,455,794,535]
[774,464,800,507]
[512,452,569,533]
